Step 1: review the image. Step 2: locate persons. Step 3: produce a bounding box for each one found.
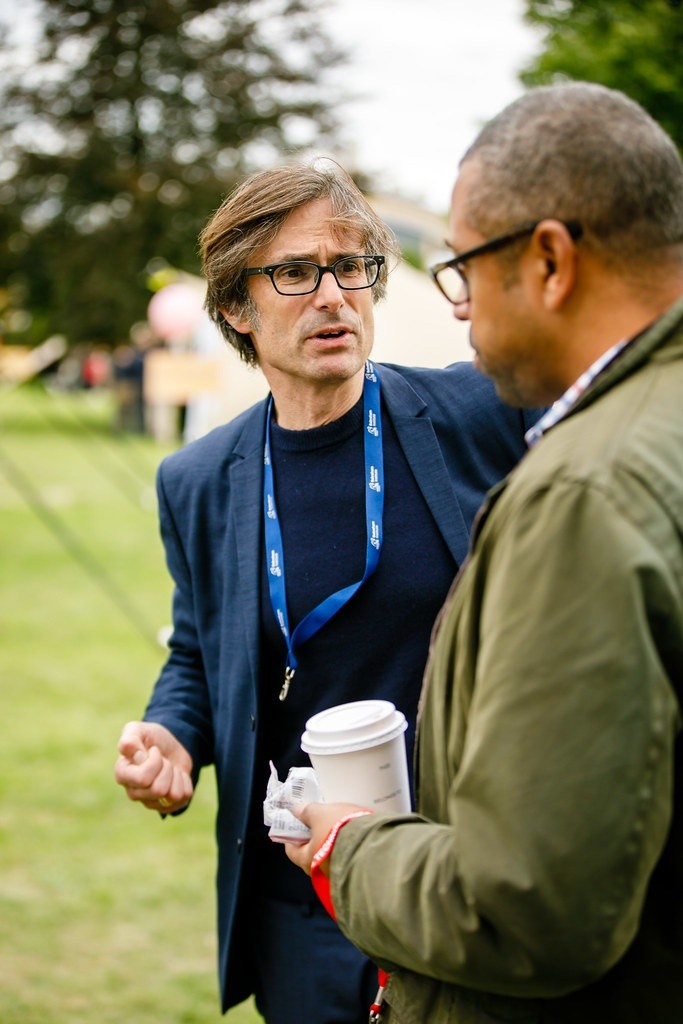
[113,159,560,1024]
[270,80,683,1023]
[6,305,196,445]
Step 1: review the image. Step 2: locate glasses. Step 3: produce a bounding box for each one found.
[427,223,578,305]
[240,254,386,297]
